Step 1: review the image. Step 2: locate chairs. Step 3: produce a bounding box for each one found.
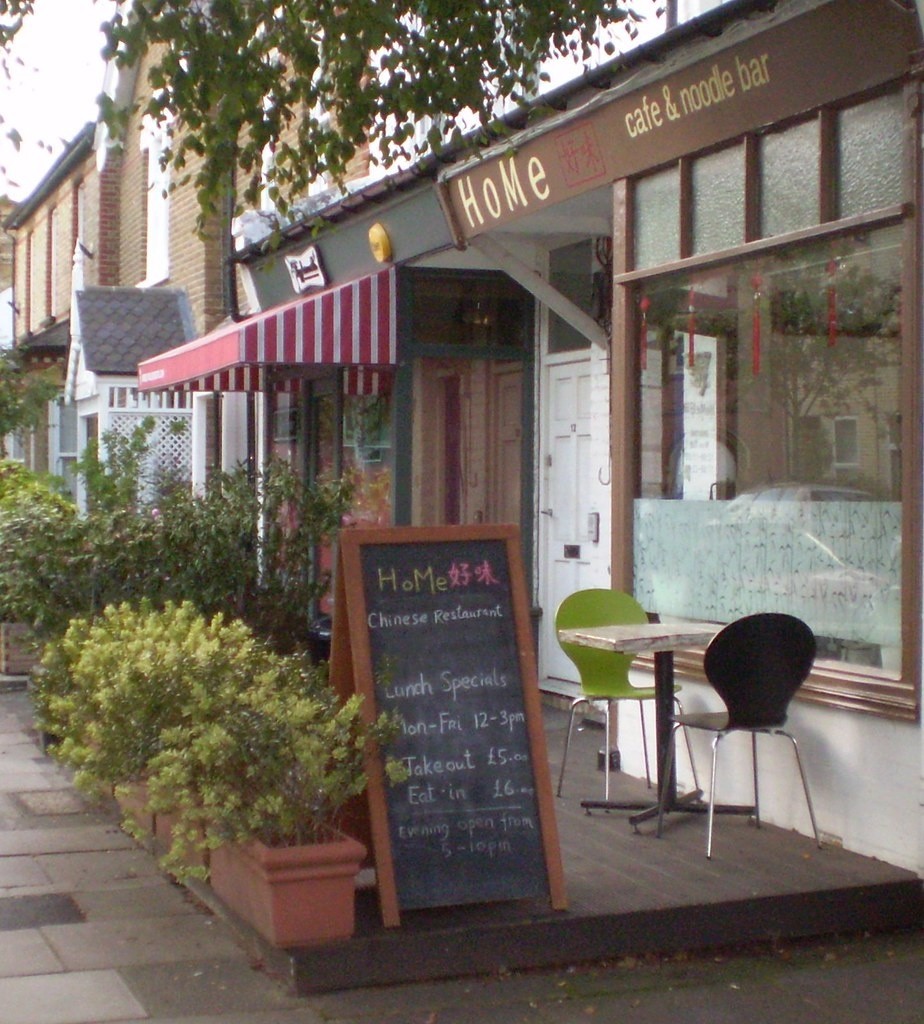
[657,612,823,861]
[554,589,702,814]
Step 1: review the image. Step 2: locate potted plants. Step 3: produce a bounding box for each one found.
[0,454,410,948]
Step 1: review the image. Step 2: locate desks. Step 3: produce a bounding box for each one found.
[558,620,760,836]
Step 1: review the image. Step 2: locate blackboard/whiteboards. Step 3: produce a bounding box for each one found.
[326,523,570,929]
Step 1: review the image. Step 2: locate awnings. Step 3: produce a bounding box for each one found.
[131,262,399,616]
